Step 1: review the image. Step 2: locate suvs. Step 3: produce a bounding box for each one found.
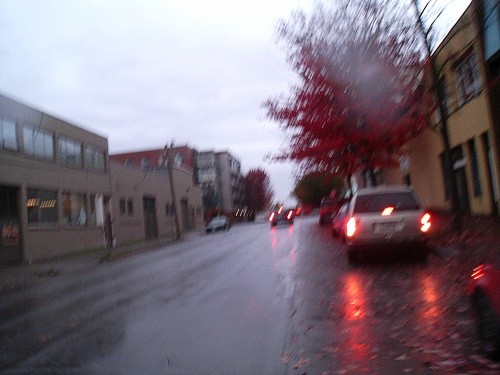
[319,200,343,223]
[345,185,429,264]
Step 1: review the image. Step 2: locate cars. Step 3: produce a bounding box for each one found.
[332,201,349,238]
[269,209,293,226]
[205,216,231,232]
